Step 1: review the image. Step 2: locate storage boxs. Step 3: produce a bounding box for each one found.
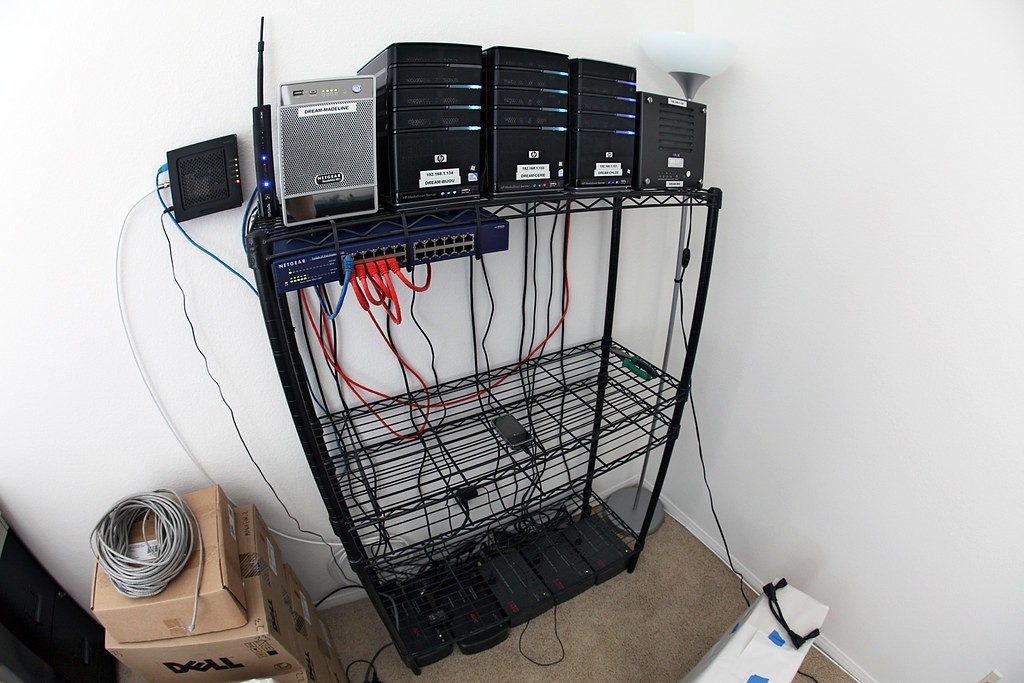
[378,514,634,667]
[88,484,351,682]
[678,577,829,683]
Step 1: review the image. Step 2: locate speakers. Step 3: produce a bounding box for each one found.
[276,75,379,227]
[478,46,571,200]
[631,89,710,190]
[360,41,485,210]
[568,58,638,194]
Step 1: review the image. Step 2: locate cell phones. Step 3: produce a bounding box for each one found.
[491,414,533,447]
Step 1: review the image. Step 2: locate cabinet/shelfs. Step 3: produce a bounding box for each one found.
[242,181,725,678]
[0,514,118,682]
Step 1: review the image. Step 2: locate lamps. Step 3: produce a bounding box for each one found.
[605,72,711,538]
